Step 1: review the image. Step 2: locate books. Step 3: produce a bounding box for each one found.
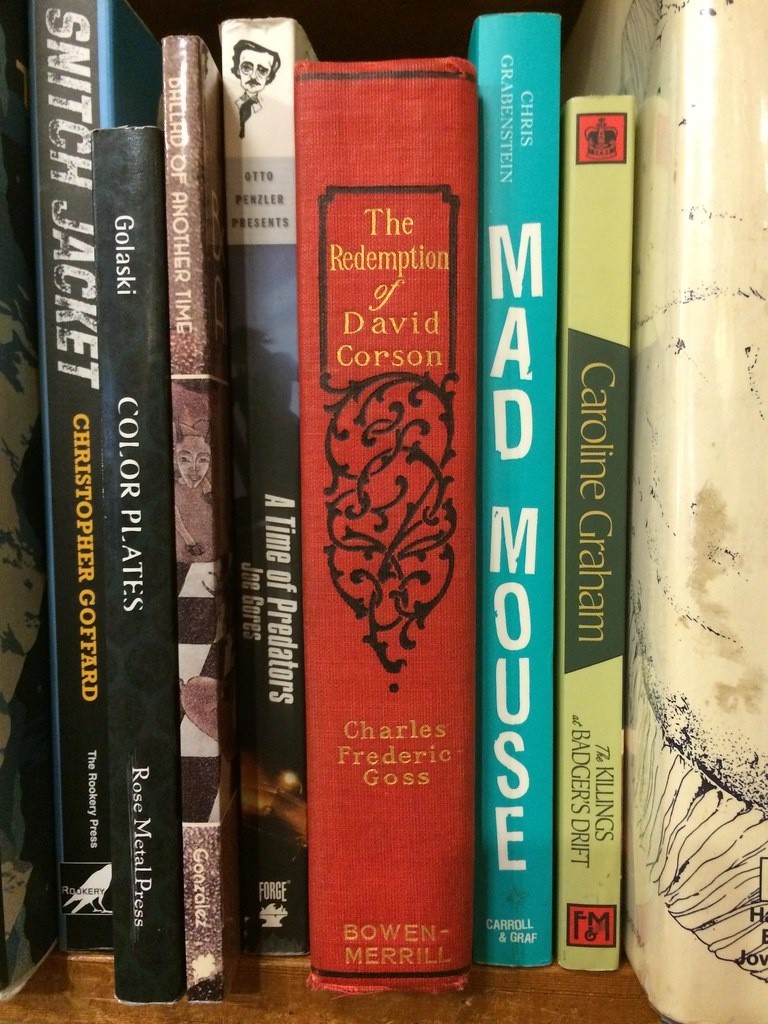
[0,1,768,1024]
